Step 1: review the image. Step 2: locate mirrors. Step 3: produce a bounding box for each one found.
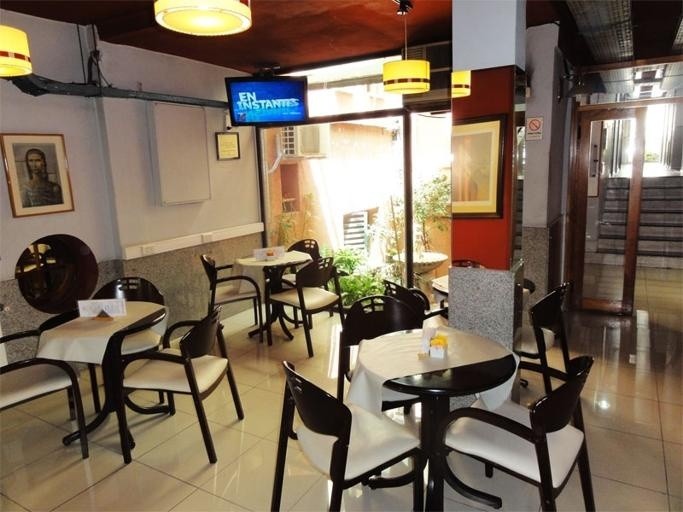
[452,65,515,271]
[506,64,527,270]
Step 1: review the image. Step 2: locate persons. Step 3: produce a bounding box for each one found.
[19,148,62,208]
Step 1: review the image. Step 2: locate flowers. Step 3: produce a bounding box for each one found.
[429,332,447,348]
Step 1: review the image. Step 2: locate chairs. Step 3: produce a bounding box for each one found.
[271,360,424,512]
[520,281,573,393]
[114,303,246,465]
[337,295,420,468]
[1,329,89,462]
[88,276,164,416]
[200,238,346,358]
[381,266,446,323]
[425,355,597,511]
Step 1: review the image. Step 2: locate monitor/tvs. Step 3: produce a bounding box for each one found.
[224,75,309,126]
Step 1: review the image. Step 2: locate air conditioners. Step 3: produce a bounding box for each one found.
[278,120,328,163]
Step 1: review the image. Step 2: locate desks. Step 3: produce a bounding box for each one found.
[44,303,171,452]
[358,326,516,512]
[432,273,535,300]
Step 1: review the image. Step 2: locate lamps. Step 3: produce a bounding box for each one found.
[450,69,472,99]
[381,1,432,97]
[0,23,34,79]
[151,0,255,36]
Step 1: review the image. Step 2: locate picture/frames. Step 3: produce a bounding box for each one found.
[216,130,242,162]
[0,132,76,218]
[450,110,510,222]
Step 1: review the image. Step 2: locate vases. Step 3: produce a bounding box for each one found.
[429,346,447,359]
